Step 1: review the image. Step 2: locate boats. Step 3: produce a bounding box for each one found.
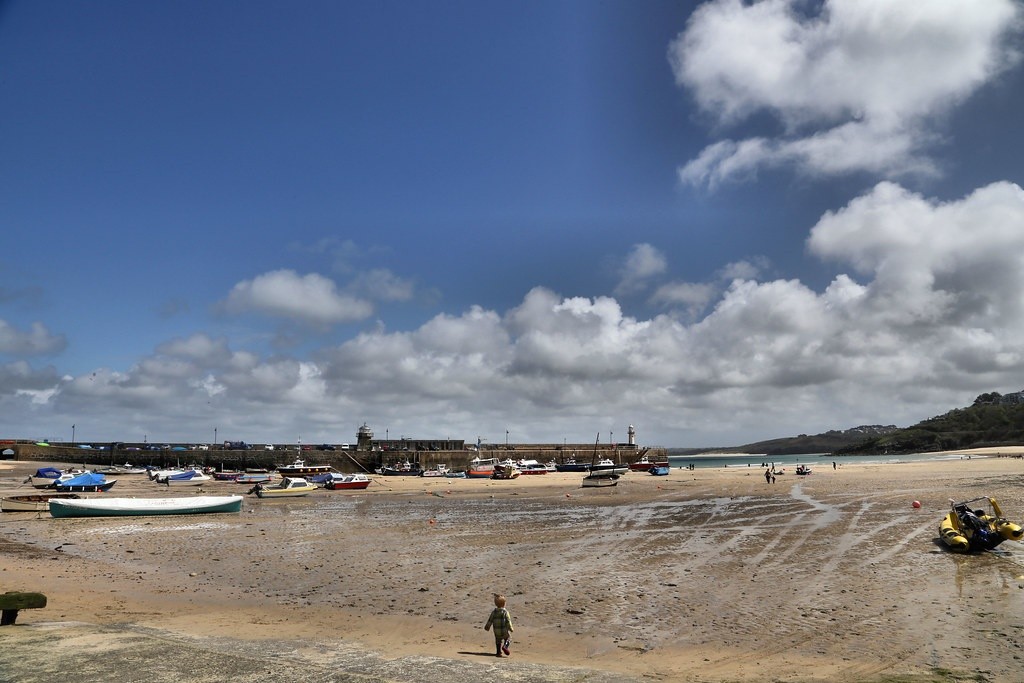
[55,473,117,493]
[773,469,785,475]
[27,466,92,488]
[795,464,813,475]
[246,477,318,498]
[92,438,671,481]
[47,495,244,519]
[326,474,373,489]
[937,496,1024,553]
[166,470,211,486]
[306,473,345,488]
[581,474,621,488]
[1,493,82,512]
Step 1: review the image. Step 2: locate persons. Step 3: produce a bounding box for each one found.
[692,464,694,470]
[762,463,765,467]
[833,461,837,470]
[771,476,775,483]
[689,463,691,470]
[484,592,515,657]
[770,462,775,473]
[764,469,771,483]
[68,467,75,473]
[765,463,768,466]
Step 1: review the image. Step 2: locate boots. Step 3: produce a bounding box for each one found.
[503,640,511,655]
[497,645,503,657]
